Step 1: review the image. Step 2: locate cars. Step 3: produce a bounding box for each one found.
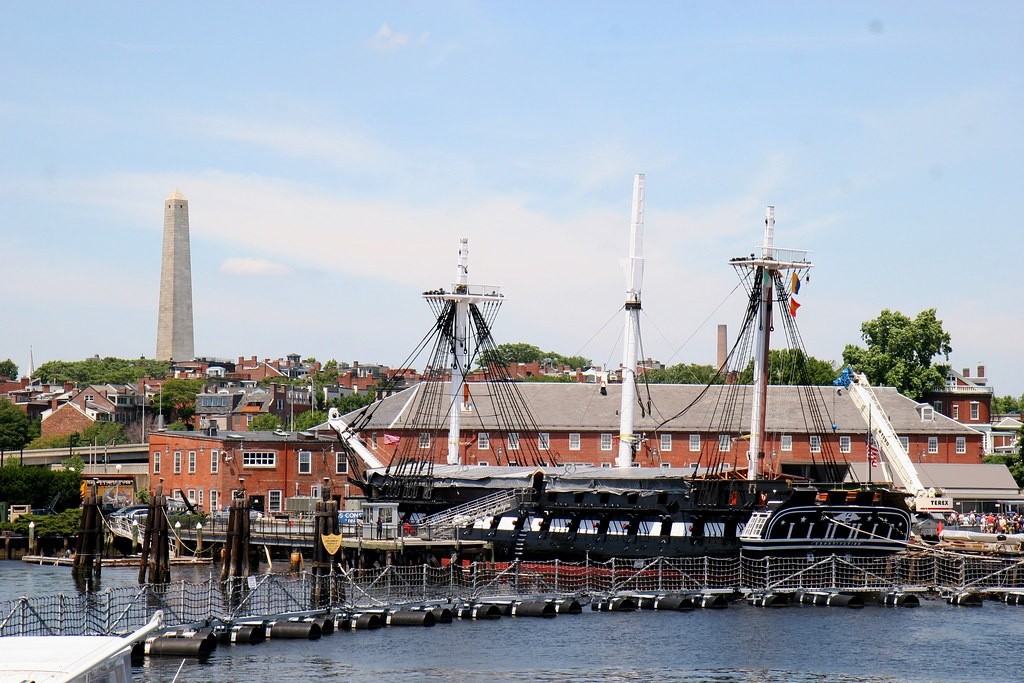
[110,505,149,520]
[215,506,258,524]
[125,508,150,525]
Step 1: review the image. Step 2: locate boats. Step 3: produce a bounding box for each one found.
[327,171,913,591]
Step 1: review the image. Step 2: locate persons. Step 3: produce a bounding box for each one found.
[377,517,383,541]
[403,519,411,538]
[65,548,71,558]
[945,509,1024,551]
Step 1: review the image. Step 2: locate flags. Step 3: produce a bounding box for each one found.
[790,272,811,318]
[384,434,399,447]
[865,431,878,467]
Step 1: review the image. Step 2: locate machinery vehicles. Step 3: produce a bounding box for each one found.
[832,364,956,518]
